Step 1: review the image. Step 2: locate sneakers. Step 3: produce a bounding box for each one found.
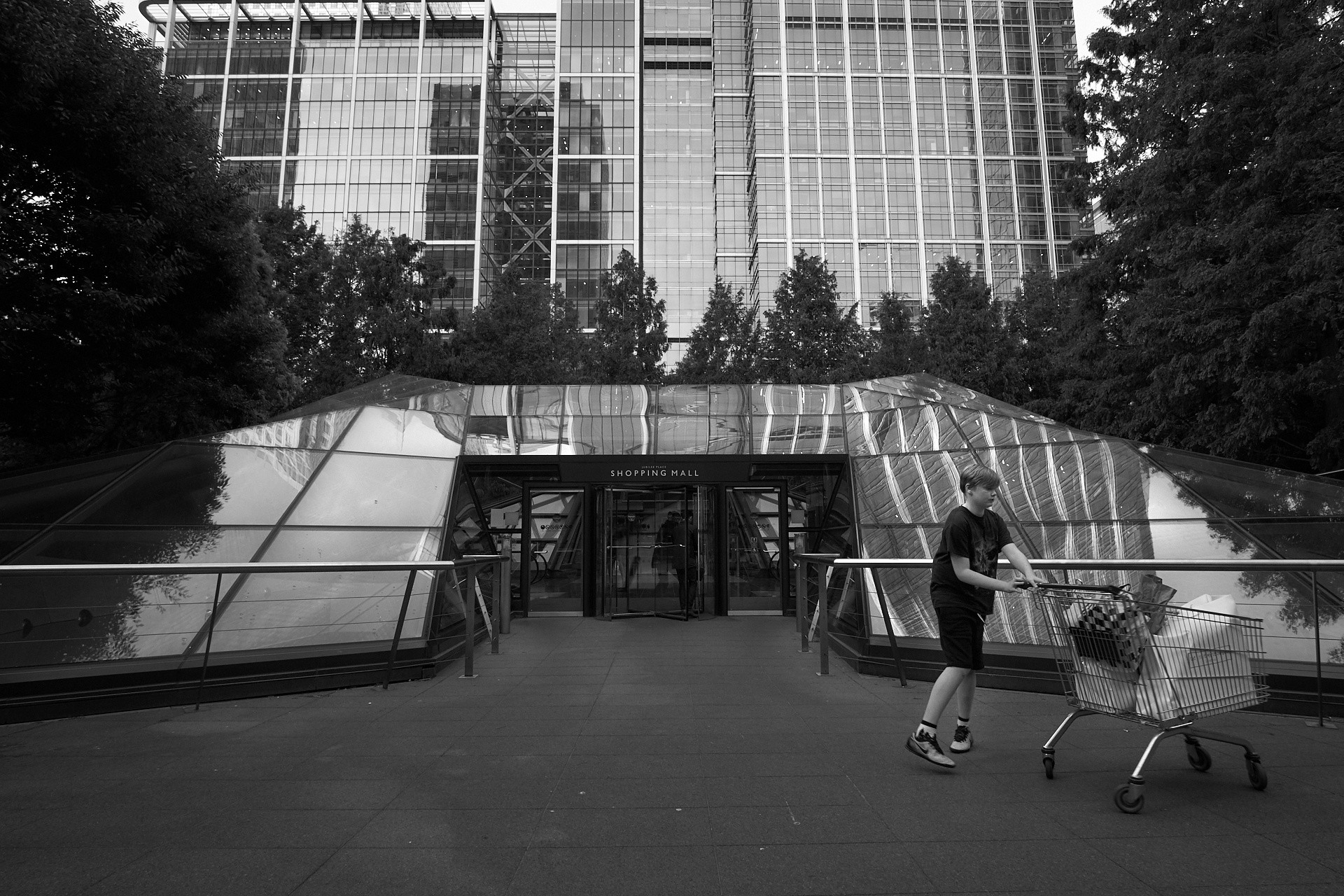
[906,731,955,768]
[950,725,974,754]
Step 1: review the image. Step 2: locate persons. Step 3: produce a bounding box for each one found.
[904,463,1050,768]
[670,509,704,618]
[650,510,682,598]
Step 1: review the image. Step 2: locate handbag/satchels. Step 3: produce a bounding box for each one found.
[1072,592,1149,672]
[1134,573,1177,634]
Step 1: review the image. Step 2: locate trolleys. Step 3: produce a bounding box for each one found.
[1015,578,1272,813]
